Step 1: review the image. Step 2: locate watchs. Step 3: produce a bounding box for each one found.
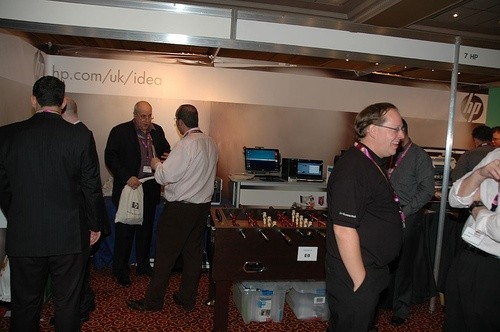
[469,201,485,215]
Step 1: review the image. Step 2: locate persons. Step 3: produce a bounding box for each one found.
[324,100,406,332]
[104,100,169,287]
[0,77,108,332]
[125,104,217,310]
[383,119,500,332]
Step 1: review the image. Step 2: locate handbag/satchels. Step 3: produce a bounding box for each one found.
[115,183,144,225]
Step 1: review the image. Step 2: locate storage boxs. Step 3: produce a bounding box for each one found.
[286,282,330,320]
[234,280,292,325]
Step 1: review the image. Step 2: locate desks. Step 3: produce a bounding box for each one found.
[208,209,327,332]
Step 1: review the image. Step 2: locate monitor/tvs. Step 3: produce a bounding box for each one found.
[245,148,279,171]
[294,160,322,179]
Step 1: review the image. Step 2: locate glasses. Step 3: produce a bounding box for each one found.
[174,118,182,122]
[372,124,404,133]
[492,137,500,141]
[135,112,154,122]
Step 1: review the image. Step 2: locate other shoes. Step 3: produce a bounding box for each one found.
[390,315,405,326]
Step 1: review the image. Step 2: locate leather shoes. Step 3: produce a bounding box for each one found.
[125,299,159,310]
[86,304,96,312]
[173,292,192,310]
[114,271,131,288]
[136,268,152,276]
[49,315,89,326]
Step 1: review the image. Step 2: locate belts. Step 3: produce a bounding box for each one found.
[458,237,499,259]
